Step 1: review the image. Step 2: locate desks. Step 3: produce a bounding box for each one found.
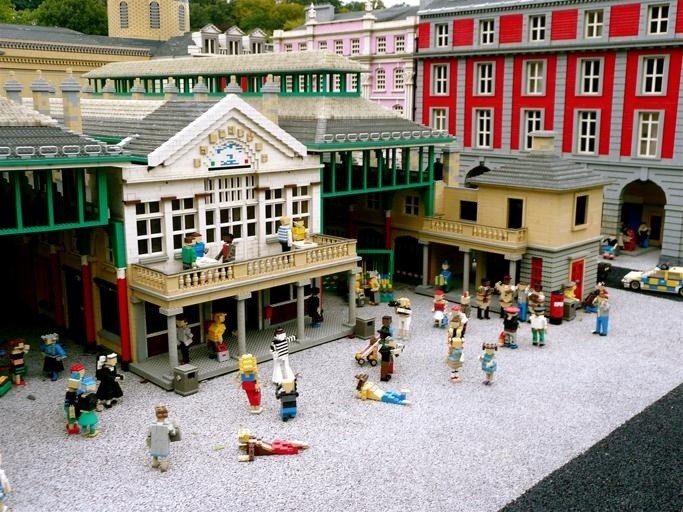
[192,256,220,268]
[291,240,317,249]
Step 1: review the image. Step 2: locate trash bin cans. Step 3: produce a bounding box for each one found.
[173,363,199,397]
[563,298,576,320]
[356,315,377,340]
